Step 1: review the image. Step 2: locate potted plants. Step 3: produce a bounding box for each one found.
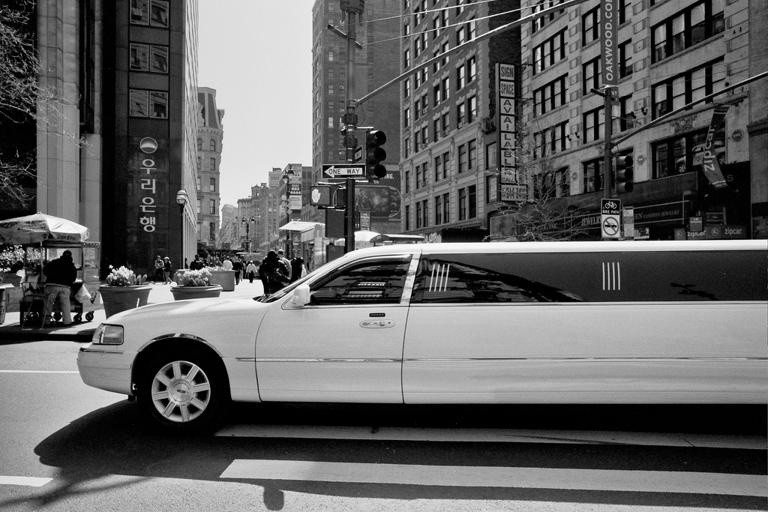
[99,264,153,319]
[170,267,224,300]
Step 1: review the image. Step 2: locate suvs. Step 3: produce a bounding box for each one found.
[675,140,725,174]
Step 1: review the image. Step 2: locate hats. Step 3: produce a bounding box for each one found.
[267,252,278,258]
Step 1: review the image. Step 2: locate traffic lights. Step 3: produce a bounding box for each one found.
[613,155,633,196]
[308,185,332,208]
[362,128,388,184]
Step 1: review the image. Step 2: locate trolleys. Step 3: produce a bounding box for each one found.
[16,240,101,328]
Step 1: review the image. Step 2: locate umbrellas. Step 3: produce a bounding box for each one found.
[0,213,90,282]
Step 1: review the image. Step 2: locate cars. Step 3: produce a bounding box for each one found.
[247,259,262,276]
[75,238,767,439]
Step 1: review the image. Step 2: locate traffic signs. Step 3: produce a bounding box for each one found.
[320,163,366,180]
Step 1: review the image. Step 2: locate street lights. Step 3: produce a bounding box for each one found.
[282,168,295,261]
[240,214,257,251]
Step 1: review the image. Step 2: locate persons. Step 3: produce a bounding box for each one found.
[162,257,172,285]
[189,249,307,296]
[153,255,164,285]
[42,250,77,327]
